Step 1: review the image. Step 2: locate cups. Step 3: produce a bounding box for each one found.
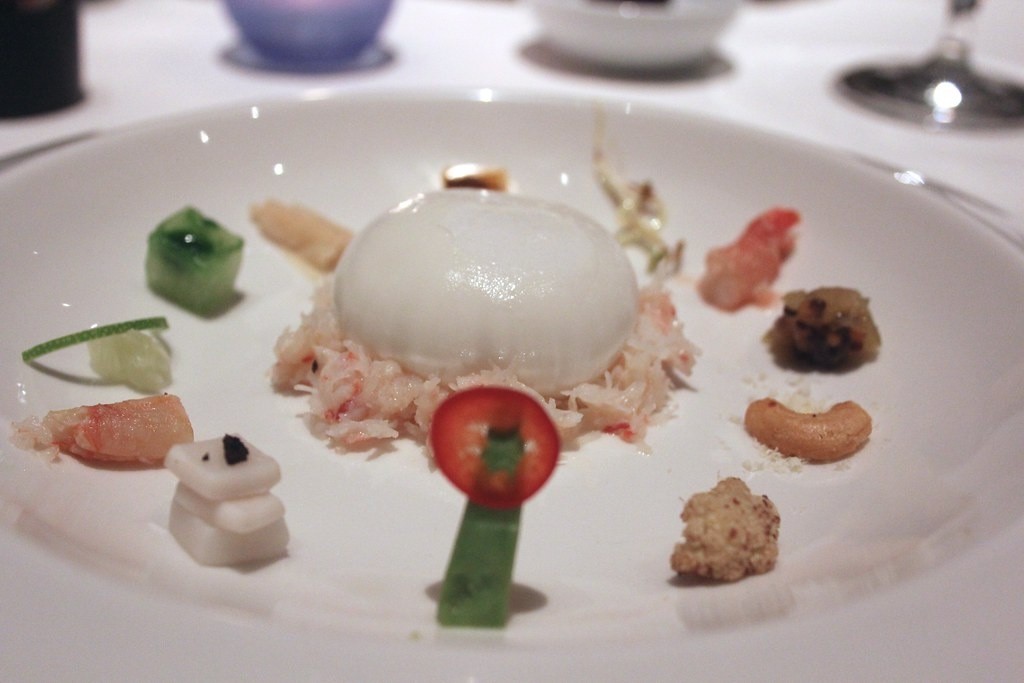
[0,0,81,119]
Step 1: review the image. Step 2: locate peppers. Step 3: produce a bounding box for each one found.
[430,384,561,510]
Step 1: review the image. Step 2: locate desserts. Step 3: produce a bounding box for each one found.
[322,184,638,405]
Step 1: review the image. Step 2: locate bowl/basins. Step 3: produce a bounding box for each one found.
[532,0,739,77]
[222,0,397,66]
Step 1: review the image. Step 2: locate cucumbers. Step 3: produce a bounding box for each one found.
[144,207,245,314]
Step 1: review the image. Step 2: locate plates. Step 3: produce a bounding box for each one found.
[0,90,1023,680]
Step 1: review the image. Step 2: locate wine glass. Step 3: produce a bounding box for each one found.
[828,0,1024,131]
